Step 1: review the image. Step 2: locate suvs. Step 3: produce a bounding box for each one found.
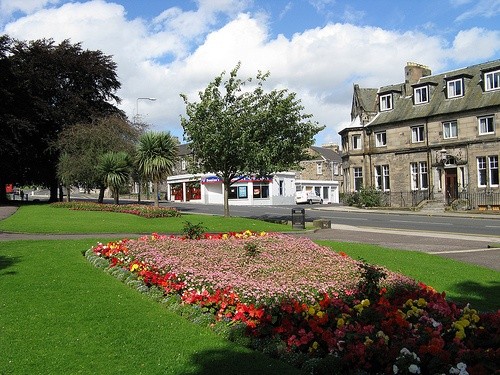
[296,190,324,205]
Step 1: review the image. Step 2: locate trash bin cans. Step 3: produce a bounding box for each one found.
[291,207,305,229]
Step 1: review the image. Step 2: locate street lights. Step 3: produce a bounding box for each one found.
[136,97,156,203]
